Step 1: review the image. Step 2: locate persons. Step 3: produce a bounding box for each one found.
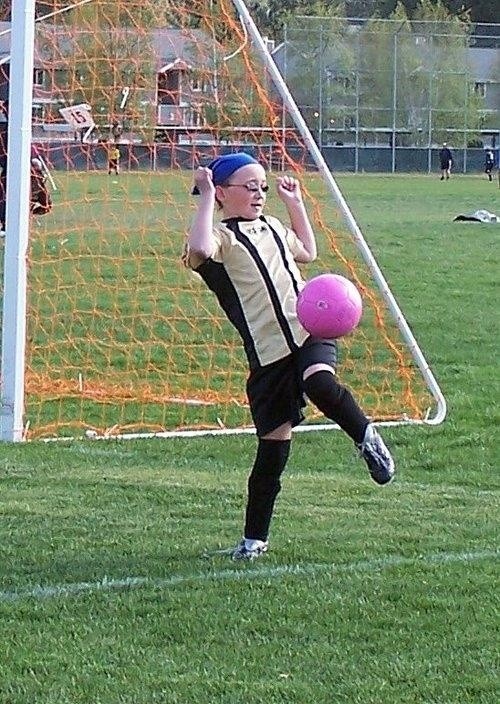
[183,151,397,559]
[483,147,496,181]
[106,142,121,176]
[490,134,497,148]
[439,141,453,181]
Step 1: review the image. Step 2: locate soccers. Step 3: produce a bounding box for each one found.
[295,273,362,339]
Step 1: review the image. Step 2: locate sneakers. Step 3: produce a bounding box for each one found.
[353,422,396,485]
[228,534,270,566]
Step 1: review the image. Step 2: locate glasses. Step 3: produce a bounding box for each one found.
[223,181,270,192]
